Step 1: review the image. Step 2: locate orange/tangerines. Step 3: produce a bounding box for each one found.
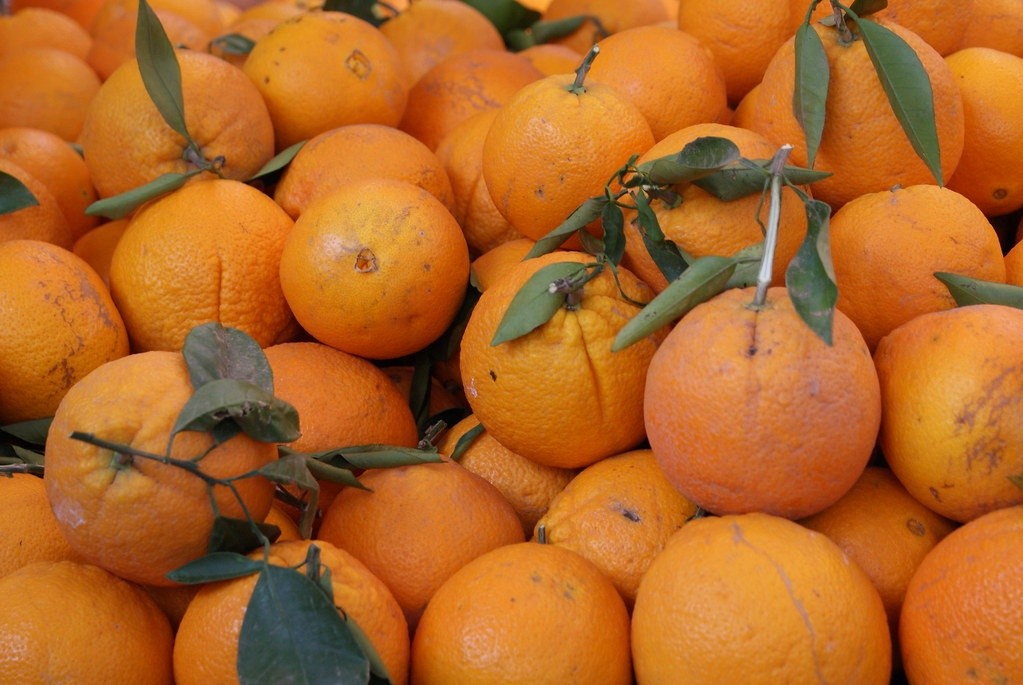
[0,0,1023,685]
